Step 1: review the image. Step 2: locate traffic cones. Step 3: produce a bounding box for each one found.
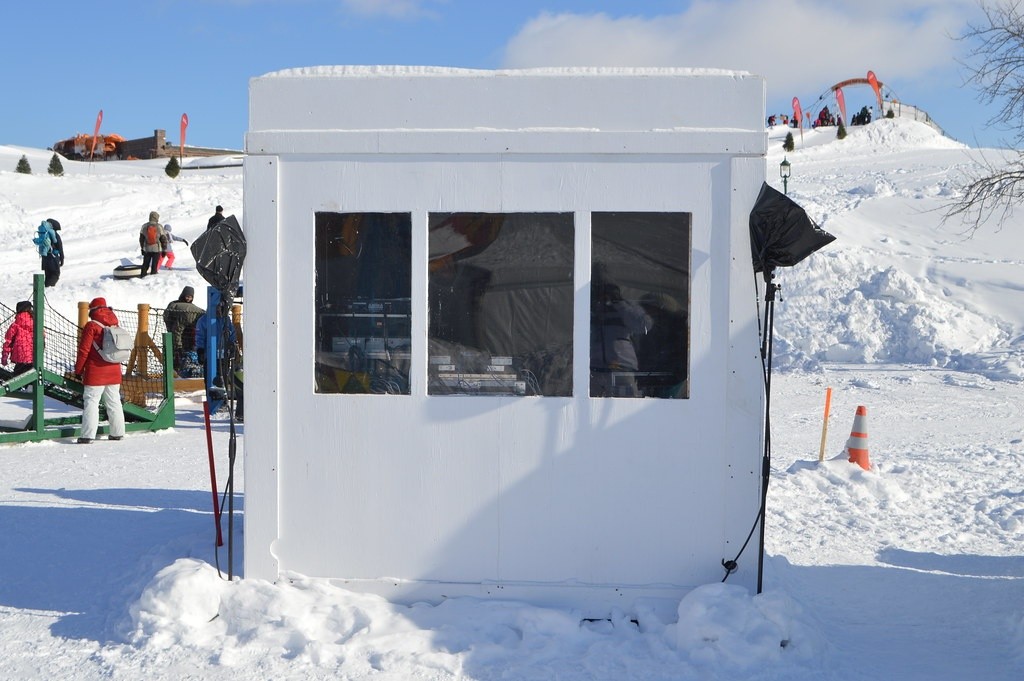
[845,406,869,471]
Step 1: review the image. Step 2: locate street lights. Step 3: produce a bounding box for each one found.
[780,155,790,195]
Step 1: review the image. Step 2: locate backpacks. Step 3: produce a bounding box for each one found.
[147,223,159,245]
[90,320,134,363]
[33,221,57,256]
[141,249,145,255]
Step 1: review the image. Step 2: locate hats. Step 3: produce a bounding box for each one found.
[216,205,223,212]
[87,298,106,309]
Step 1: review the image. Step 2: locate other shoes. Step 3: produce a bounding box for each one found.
[108,435,123,440]
[165,266,172,270]
[45,284,55,287]
[151,272,158,274]
[77,437,93,443]
[140,274,147,279]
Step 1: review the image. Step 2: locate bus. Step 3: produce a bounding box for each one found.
[318,313,411,355]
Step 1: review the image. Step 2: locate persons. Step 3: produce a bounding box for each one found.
[850,106,873,126]
[549,261,654,398]
[768,114,777,126]
[163,286,235,396]
[207,205,225,229]
[1,301,46,390]
[780,114,788,124]
[157,224,188,272]
[138,211,168,279]
[41,218,64,287]
[793,111,803,128]
[74,297,125,444]
[829,113,842,126]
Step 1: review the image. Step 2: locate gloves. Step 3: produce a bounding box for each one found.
[162,251,166,257]
[183,239,188,246]
[75,374,82,381]
[197,348,205,364]
[59,260,63,266]
[1,355,7,366]
